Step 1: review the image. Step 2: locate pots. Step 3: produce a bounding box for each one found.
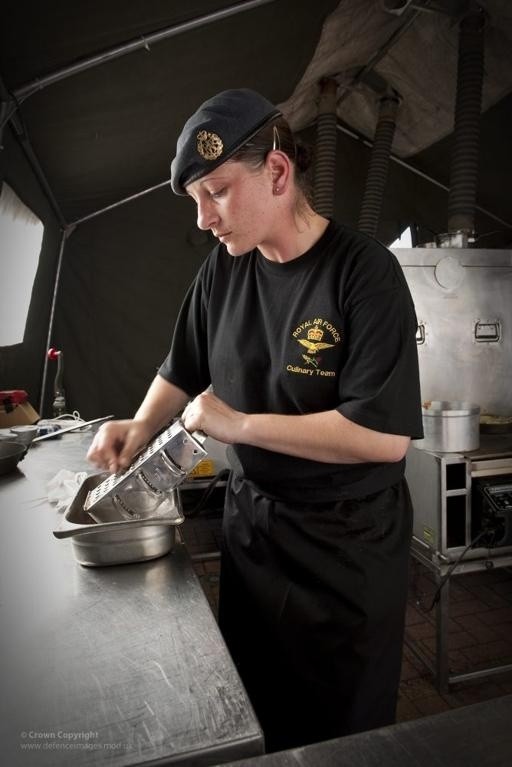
[411,399,487,453]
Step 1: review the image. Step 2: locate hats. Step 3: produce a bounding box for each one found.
[170,88,283,198]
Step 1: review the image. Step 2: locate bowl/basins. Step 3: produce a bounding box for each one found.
[52,467,187,567]
[0,441,29,474]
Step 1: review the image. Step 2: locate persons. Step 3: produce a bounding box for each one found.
[84,87,425,756]
